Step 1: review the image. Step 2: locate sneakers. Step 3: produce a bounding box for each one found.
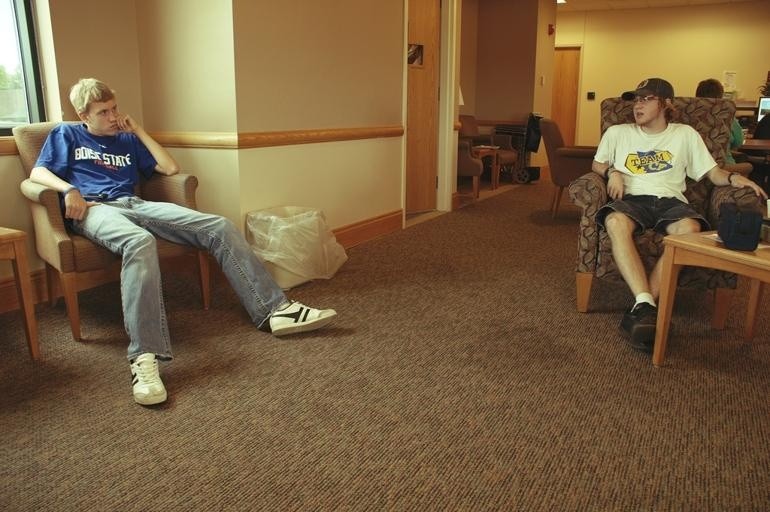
[127,351,168,407]
[617,307,656,352]
[267,298,338,335]
[628,300,678,345]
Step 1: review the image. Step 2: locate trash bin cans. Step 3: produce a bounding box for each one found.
[244,206,329,292]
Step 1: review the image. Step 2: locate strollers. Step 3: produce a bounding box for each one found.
[481,113,542,185]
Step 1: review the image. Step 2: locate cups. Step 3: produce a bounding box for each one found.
[742,128,749,145]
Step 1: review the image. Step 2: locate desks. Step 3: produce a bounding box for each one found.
[734,99,760,115]
[651,229,770,365]
[473,146,501,191]
[735,110,756,125]
[736,137,770,166]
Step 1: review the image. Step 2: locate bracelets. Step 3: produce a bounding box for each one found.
[63,186,80,197]
[604,167,619,177]
[729,173,735,183]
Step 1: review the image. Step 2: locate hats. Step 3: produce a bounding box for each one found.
[621,77,675,101]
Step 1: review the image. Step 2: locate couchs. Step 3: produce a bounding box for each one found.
[458,136,484,199]
[539,118,599,221]
[569,96,762,329]
[459,114,519,188]
[12,121,210,340]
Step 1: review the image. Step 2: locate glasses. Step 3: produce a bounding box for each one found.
[631,96,664,106]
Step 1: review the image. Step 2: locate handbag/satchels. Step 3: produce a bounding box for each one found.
[716,199,763,251]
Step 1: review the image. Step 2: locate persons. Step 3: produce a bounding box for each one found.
[753,112,770,140]
[29,77,337,406]
[592,78,769,351]
[696,79,744,147]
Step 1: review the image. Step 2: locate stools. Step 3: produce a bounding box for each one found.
[0,227,41,362]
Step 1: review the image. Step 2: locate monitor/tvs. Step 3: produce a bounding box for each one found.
[756,96,770,123]
